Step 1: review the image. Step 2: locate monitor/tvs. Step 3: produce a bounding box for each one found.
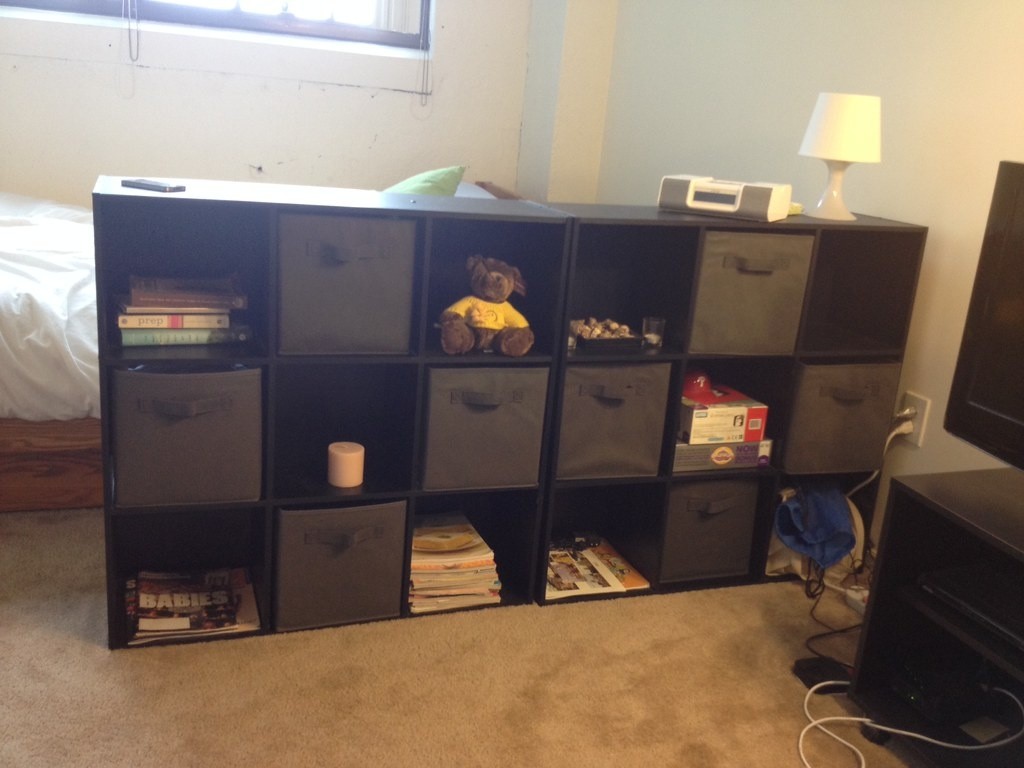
[942,161,1024,471]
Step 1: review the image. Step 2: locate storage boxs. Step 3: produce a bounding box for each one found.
[665,435,770,472]
[676,383,769,445]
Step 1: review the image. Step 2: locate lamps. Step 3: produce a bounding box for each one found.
[799,88,882,222]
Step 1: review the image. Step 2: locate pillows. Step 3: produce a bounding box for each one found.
[376,165,466,194]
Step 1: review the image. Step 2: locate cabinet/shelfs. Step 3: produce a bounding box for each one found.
[90,174,931,652]
[849,469,1024,768]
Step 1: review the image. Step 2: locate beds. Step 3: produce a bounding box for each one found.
[0,176,522,511]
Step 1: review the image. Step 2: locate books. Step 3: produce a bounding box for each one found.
[407,511,502,614]
[125,566,261,644]
[115,273,250,347]
[544,527,651,600]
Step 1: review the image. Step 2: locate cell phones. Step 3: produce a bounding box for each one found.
[121,179,185,193]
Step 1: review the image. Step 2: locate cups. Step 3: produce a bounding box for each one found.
[642,316,665,348]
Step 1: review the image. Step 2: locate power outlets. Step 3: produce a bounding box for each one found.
[893,390,930,448]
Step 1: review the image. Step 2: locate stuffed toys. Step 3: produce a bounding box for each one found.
[441,255,536,357]
